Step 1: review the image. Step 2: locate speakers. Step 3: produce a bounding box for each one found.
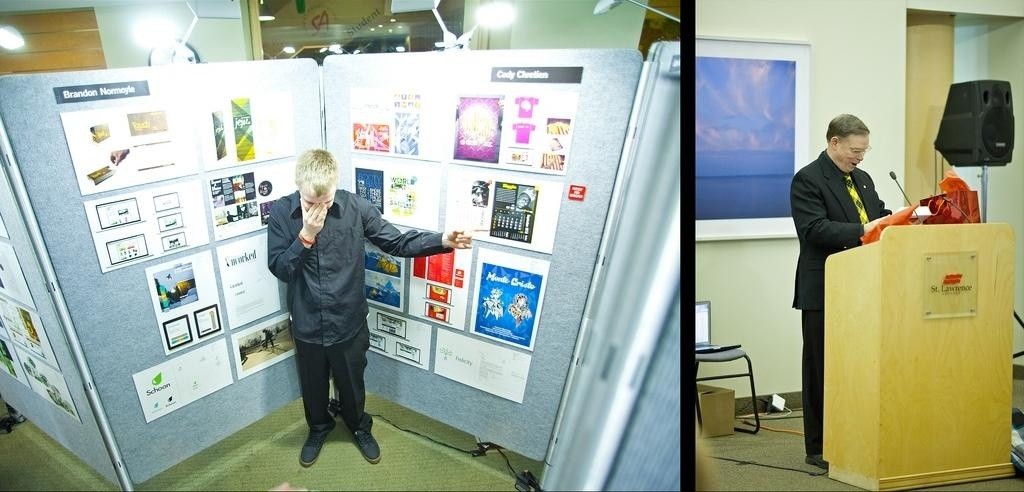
[934,79,1014,167]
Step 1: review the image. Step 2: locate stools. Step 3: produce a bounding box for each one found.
[695,350,763,440]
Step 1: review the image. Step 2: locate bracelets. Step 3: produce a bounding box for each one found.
[298,233,315,244]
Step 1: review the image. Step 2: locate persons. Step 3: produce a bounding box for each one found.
[249,202,258,217]
[790,114,892,470]
[268,149,472,468]
[238,204,248,220]
[227,211,238,222]
[263,329,273,348]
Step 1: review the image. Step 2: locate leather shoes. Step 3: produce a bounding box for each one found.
[805,455,828,470]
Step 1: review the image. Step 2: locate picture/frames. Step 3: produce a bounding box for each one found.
[693,34,812,246]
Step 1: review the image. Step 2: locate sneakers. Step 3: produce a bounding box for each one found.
[354,431,381,464]
[300,440,323,467]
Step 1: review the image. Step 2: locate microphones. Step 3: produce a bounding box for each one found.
[885,171,922,226]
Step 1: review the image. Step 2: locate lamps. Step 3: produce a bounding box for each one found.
[590,0,680,28]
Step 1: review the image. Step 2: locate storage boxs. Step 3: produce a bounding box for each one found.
[697,384,735,440]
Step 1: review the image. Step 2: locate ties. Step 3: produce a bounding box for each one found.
[843,174,870,225]
